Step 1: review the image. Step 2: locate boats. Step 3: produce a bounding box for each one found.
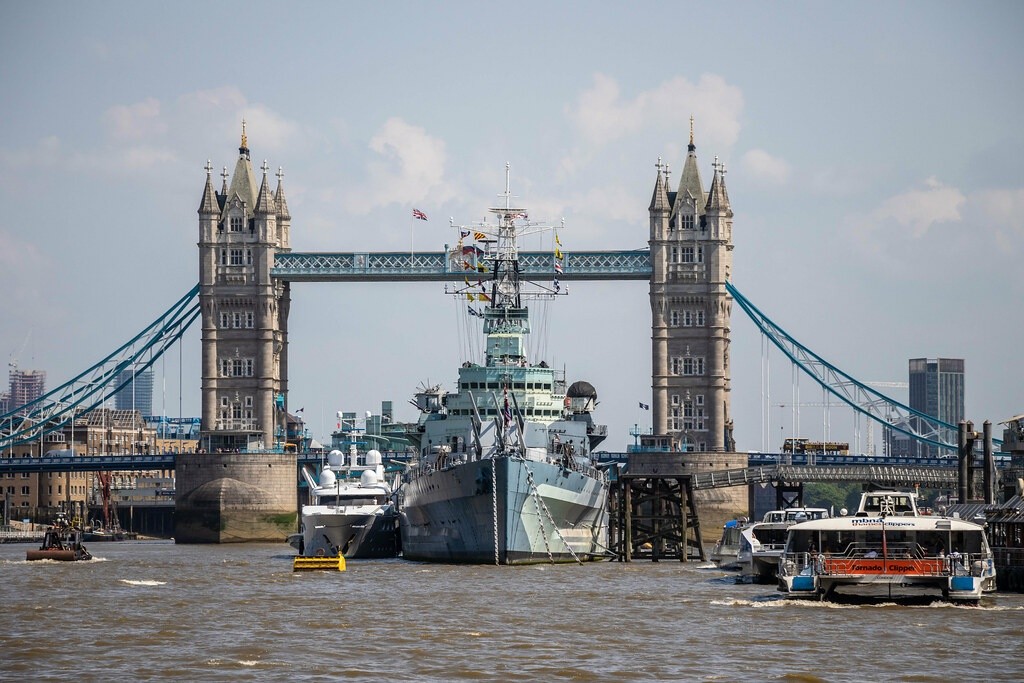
[298,450,402,562]
[734,507,827,587]
[711,517,756,571]
[777,514,997,605]
[24,517,93,563]
[398,203,612,566]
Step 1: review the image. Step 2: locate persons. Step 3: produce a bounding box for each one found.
[938,548,963,559]
[864,547,878,558]
[903,546,912,558]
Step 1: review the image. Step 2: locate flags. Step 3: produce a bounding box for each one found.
[553,234,564,294]
[459,229,493,318]
[639,401,649,410]
[413,208,428,221]
